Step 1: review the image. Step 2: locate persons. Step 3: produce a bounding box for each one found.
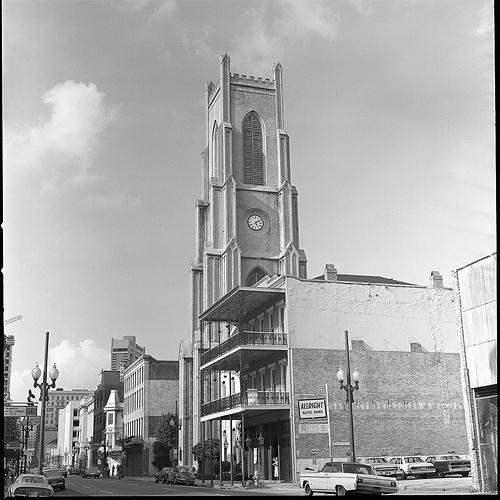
[272,457,278,477]
[112,465,115,476]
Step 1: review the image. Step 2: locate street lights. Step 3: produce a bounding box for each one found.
[337,331,359,463]
[31,332,59,473]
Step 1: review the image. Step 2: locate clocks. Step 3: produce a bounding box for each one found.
[247,214,264,231]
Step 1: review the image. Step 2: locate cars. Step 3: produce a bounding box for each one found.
[82,466,101,478]
[299,453,471,497]
[154,466,196,486]
[8,467,67,497]
